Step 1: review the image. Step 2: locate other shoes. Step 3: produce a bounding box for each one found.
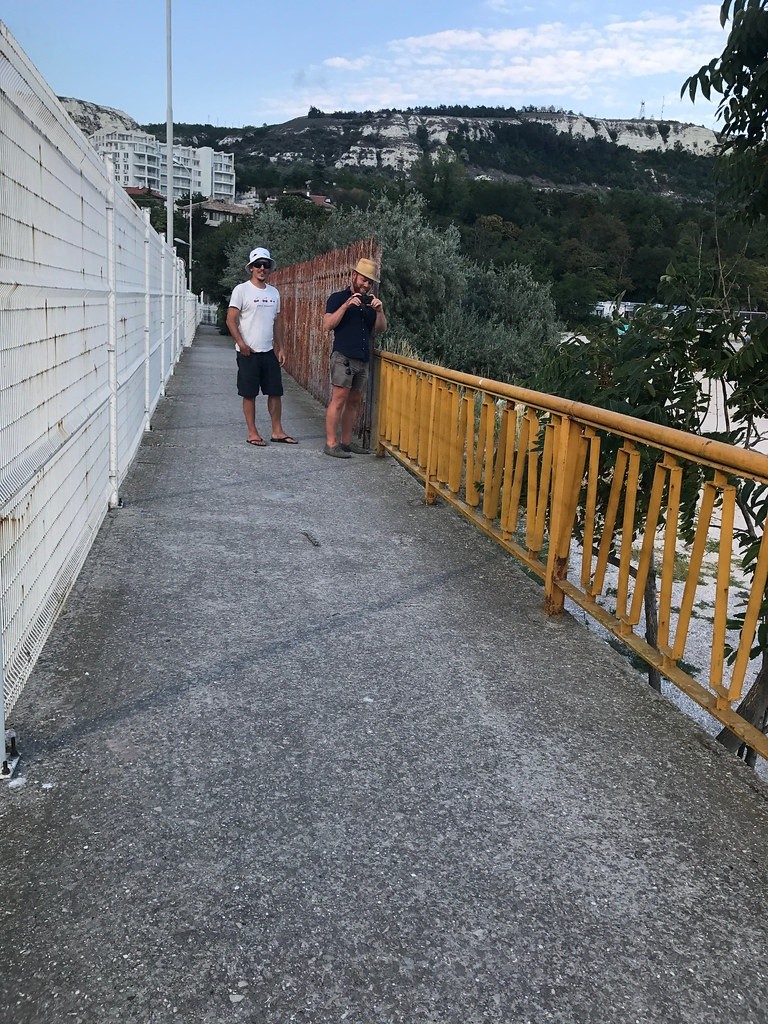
[339,440,369,454]
[324,443,351,458]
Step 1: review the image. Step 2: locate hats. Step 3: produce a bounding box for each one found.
[351,258,382,283]
[245,248,277,275]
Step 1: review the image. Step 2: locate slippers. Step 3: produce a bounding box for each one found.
[271,434,298,444]
[246,437,267,446]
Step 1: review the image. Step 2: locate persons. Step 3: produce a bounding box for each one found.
[323,259,388,459]
[227,248,298,448]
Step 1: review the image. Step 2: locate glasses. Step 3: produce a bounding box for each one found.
[253,260,271,269]
[343,360,351,376]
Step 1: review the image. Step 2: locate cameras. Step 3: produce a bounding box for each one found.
[359,296,373,304]
